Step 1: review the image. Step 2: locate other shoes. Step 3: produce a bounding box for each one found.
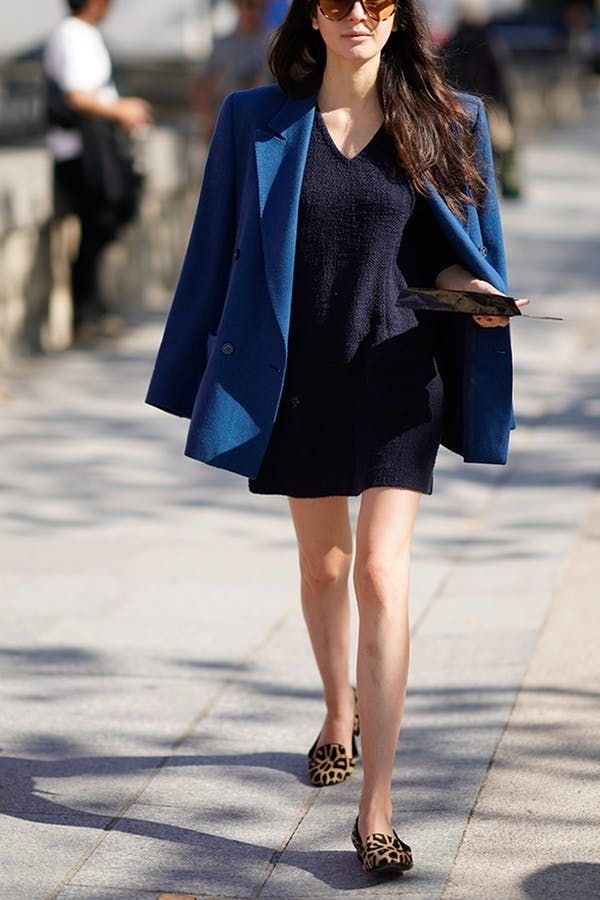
[307,687,360,786]
[351,813,413,873]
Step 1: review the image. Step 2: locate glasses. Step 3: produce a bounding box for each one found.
[316,0,397,21]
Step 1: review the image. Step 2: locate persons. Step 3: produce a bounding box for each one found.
[146,0,514,873]
[196,0,519,199]
[40,0,154,337]
